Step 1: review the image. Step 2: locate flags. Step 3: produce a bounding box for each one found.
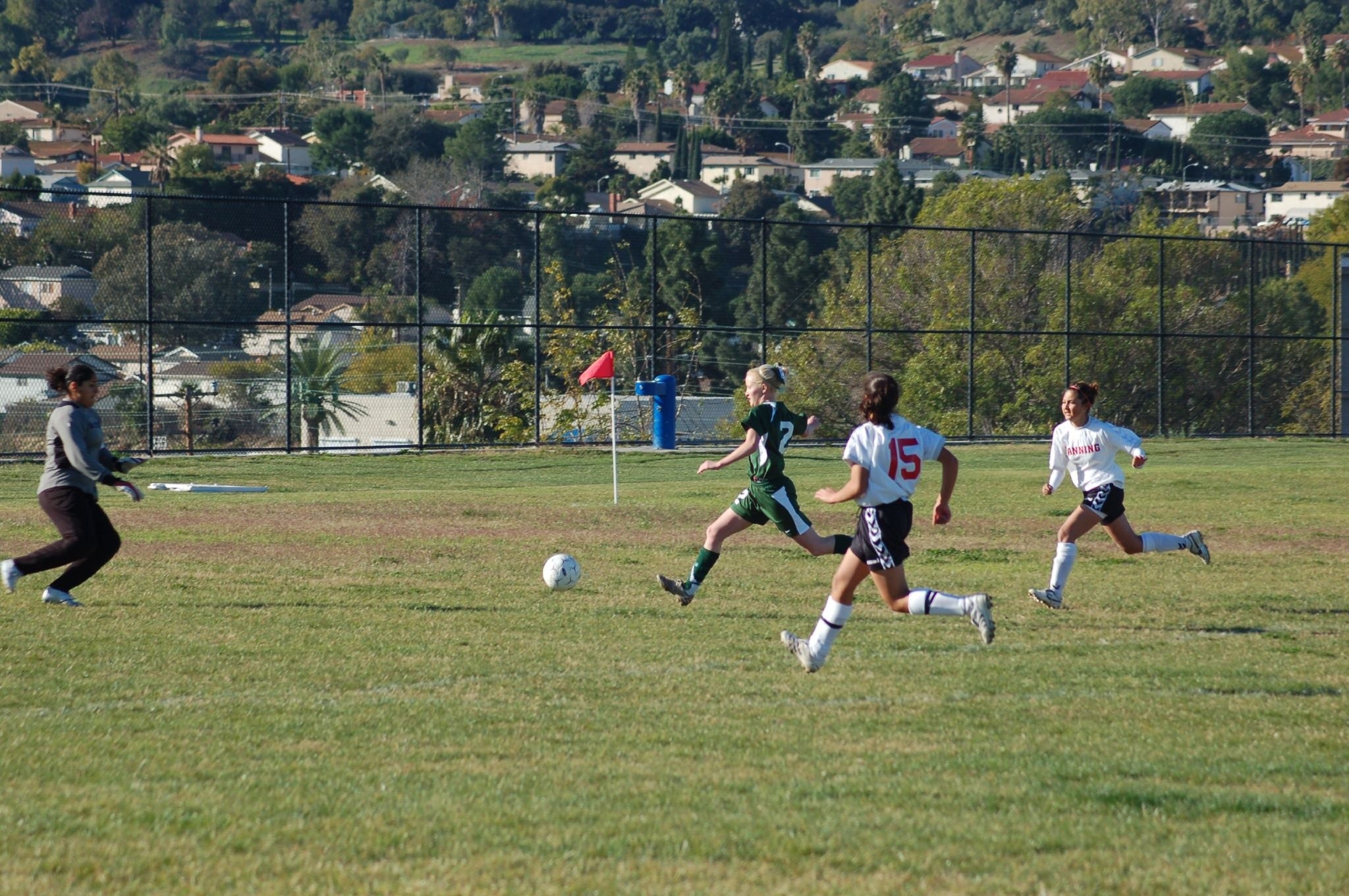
[578,350,614,386]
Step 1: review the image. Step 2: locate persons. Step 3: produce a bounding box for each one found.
[780,373,996,673]
[1027,381,1211,609]
[1,364,145,609]
[657,362,854,607]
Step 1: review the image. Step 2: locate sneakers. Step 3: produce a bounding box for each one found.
[656,574,695,607]
[42,586,83,607]
[1182,530,1210,566]
[1028,587,1062,610]
[1,559,20,594]
[781,631,824,674]
[966,594,996,644]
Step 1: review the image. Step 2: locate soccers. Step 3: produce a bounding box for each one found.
[543,553,580,591]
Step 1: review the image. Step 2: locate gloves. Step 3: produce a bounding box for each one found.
[118,457,146,474]
[113,481,144,503]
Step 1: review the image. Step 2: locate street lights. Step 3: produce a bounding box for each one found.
[775,142,791,162]
[310,86,324,97]
[597,175,610,193]
[1183,163,1199,182]
[491,75,504,85]
[1309,138,1326,182]
